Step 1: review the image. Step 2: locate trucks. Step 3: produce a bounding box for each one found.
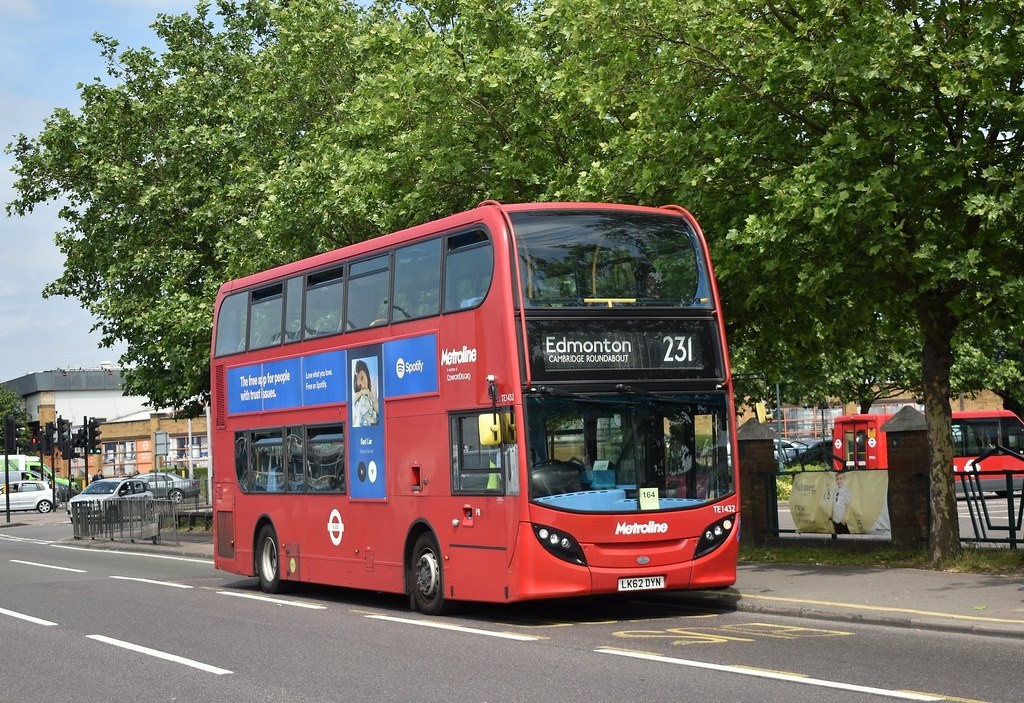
[0,454,83,502]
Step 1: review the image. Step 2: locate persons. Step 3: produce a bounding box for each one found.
[828,473,852,534]
[632,265,667,300]
[92,470,104,482]
[496,427,542,482]
[355,360,379,429]
[22,475,28,480]
[237,454,345,491]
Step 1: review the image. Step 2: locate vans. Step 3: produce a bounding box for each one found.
[0,469,59,497]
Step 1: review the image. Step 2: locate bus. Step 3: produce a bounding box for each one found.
[209,200,774,616]
[833,409,1024,498]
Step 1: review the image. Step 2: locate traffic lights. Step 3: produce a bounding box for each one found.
[28,421,39,437]
[78,430,87,447]
[3,416,22,451]
[89,416,106,454]
[31,437,37,444]
[57,419,69,451]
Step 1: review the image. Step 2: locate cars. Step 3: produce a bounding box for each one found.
[66,478,155,524]
[0,480,57,514]
[127,471,200,504]
[725,436,833,471]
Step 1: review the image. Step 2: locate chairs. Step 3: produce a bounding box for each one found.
[237,306,404,351]
